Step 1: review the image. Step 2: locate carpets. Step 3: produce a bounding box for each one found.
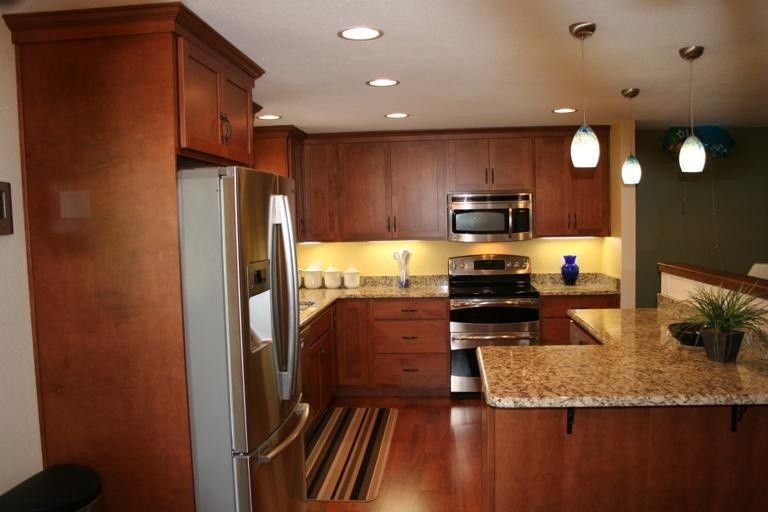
[304,405,400,504]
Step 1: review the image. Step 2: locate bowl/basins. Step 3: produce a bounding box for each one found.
[298,268,302,288]
[302,271,323,288]
[342,272,360,288]
[668,324,704,347]
[325,272,342,288]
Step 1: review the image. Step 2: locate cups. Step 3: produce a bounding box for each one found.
[400,263,409,287]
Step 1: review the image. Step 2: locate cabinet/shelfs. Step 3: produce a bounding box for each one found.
[447,127,535,193]
[534,124,612,238]
[541,296,620,347]
[303,129,447,242]
[336,297,450,400]
[297,302,335,449]
[254,124,303,242]
[0,2,265,167]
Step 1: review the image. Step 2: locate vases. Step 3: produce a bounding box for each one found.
[562,255,579,286]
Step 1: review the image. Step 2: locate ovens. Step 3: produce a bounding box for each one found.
[450,299,540,395]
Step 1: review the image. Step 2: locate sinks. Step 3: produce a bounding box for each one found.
[299,300,314,321]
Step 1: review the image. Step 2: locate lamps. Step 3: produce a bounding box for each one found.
[679,46,707,174]
[570,22,601,167]
[621,88,642,186]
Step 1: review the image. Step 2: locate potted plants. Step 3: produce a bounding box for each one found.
[675,280,764,364]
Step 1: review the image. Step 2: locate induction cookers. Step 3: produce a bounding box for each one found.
[450,281,538,298]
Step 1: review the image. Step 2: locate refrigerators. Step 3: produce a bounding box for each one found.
[177,165,311,511]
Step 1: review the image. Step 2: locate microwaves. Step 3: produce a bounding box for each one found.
[447,193,532,242]
